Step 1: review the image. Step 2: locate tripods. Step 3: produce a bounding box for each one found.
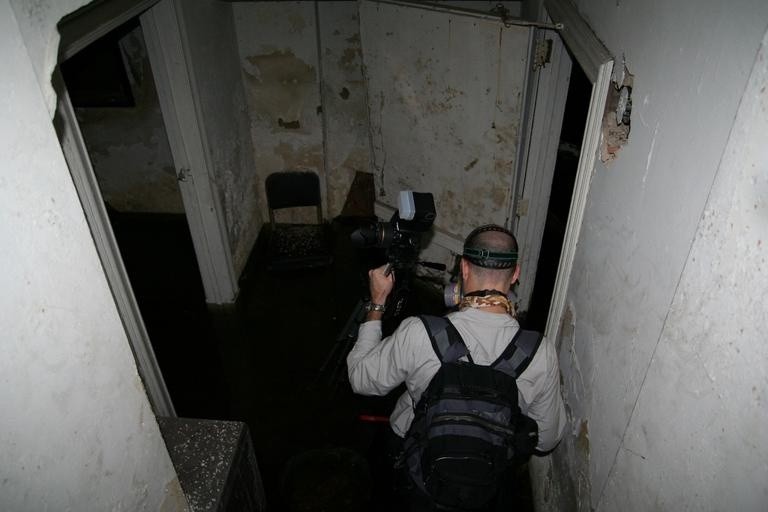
[308,259,407,401]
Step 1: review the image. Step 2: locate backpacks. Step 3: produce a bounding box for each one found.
[392,313,543,512]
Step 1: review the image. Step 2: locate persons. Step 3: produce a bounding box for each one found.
[345,224,568,511]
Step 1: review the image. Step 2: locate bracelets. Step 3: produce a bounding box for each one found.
[364,303,386,312]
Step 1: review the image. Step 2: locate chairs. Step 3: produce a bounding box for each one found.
[265,171,322,231]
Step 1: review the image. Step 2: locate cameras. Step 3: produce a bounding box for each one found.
[375,189,436,260]
